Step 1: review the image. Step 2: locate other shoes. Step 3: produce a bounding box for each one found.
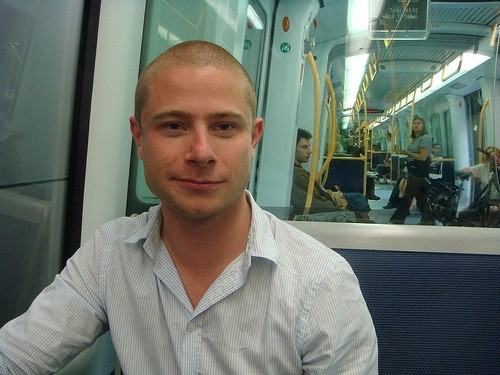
[383,202,397,209]
[417,219,435,225]
[366,193,380,200]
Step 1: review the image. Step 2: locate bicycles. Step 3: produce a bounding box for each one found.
[429,150,500,228]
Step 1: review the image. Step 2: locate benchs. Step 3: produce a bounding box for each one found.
[322,150,454,193]
[283,221,500,375]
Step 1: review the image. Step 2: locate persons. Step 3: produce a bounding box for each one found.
[389,115,436,225]
[457,150,500,229]
[291,128,375,223]
[1,41,379,375]
[335,127,444,210]
